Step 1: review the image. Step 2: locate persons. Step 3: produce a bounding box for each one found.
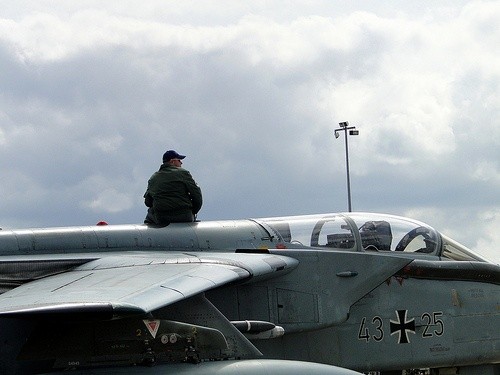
[142,149,204,228]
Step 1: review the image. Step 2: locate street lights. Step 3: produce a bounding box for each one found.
[334,121,359,213]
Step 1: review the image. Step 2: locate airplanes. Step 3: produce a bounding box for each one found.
[1,211,499,374]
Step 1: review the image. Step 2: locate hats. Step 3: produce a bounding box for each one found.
[163,150,186,163]
